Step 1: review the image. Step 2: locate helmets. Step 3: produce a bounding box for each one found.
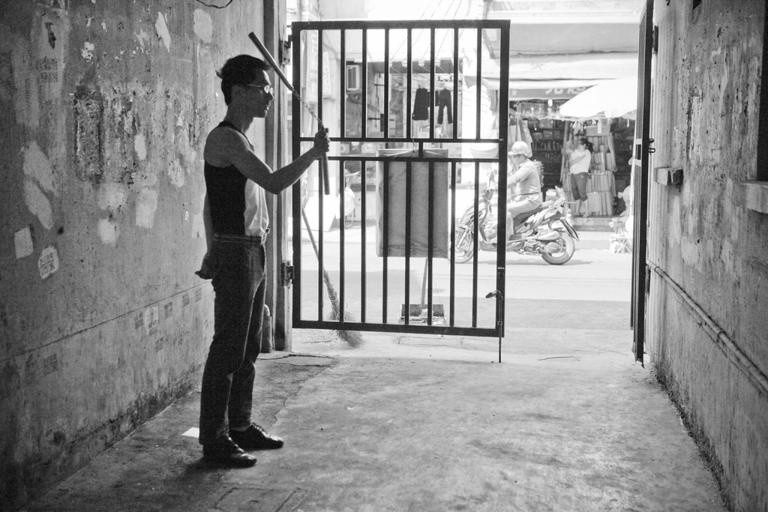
[508,141,532,157]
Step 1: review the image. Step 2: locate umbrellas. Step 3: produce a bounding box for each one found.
[560,80,637,217]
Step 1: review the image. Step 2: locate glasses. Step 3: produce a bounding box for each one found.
[243,84,273,96]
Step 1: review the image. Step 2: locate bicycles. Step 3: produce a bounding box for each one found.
[343,168,361,229]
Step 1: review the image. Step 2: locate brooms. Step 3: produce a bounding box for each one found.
[301,207,364,347]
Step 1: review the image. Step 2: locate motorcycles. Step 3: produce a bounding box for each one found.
[447,166,580,265]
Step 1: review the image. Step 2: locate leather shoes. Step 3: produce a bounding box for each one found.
[203,423,284,466]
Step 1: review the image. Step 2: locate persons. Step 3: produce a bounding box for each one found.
[194,55,330,466]
[570,137,592,217]
[486,140,543,246]
